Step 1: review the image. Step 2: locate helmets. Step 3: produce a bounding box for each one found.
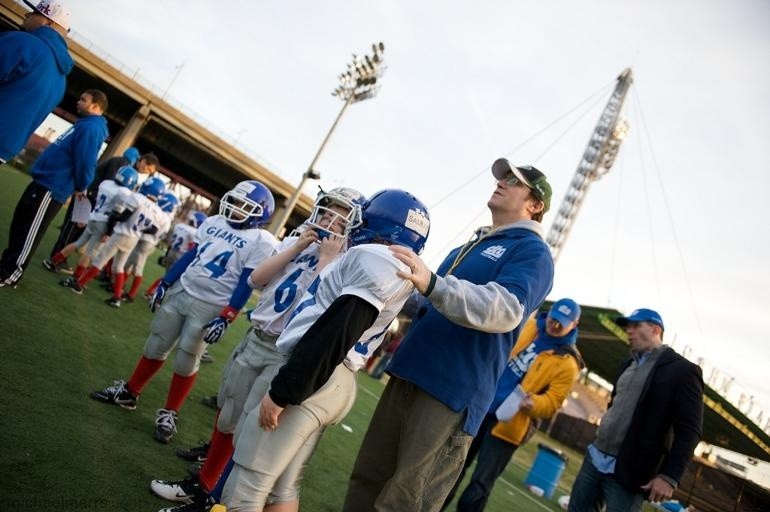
[124,147,141,167]
[115,166,138,190]
[306,186,366,244]
[219,180,275,229]
[344,189,430,254]
[188,212,207,228]
[137,177,165,202]
[157,193,178,212]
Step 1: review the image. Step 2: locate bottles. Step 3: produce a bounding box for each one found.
[529,484,544,496]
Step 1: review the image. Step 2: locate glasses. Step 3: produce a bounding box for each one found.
[499,175,531,192]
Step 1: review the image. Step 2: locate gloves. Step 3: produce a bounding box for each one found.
[203,314,230,344]
[149,280,169,312]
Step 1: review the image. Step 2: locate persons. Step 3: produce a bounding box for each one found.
[43,148,180,306]
[342,158,554,511]
[567,309,704,511]
[440,299,585,511]
[1,88,111,291]
[2,1,76,168]
[88,185,429,510]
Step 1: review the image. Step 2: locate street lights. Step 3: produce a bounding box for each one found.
[272,37,389,241]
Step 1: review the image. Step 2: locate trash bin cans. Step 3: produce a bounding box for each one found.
[524,442,570,502]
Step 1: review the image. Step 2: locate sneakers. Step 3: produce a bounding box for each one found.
[202,396,217,409]
[43,257,60,272]
[90,379,137,411]
[59,277,88,294]
[121,293,133,303]
[176,440,209,477]
[151,475,211,505]
[104,298,121,308]
[158,497,216,512]
[95,270,123,294]
[153,409,179,444]
[56,261,74,274]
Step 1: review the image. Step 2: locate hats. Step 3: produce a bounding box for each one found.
[22,0,71,30]
[617,309,664,330]
[492,158,552,213]
[548,298,581,327]
